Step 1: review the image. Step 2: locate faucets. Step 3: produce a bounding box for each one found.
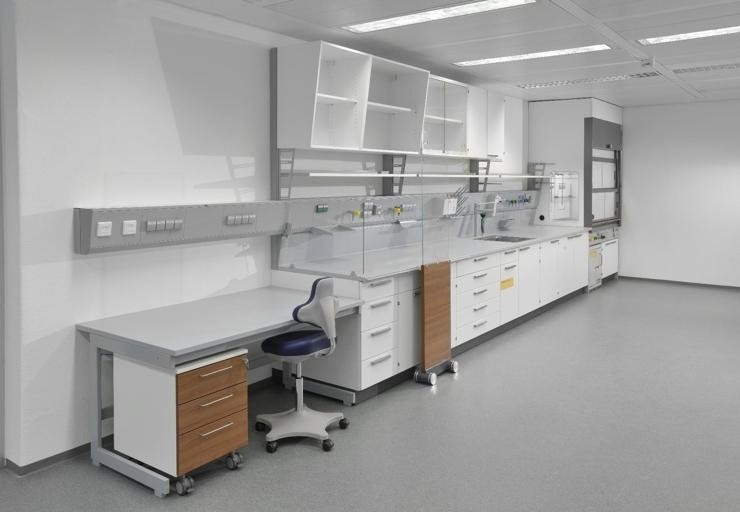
[480,212,489,236]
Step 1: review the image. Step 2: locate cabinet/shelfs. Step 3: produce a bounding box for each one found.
[469,83,507,162]
[271,227,593,407]
[528,98,624,296]
[275,40,430,156]
[426,72,470,157]
[113,348,249,496]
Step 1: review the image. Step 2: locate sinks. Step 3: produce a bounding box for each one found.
[471,234,536,242]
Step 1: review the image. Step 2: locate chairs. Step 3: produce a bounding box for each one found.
[255,277,349,453]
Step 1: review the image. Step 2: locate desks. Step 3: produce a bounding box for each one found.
[76,286,364,498]
